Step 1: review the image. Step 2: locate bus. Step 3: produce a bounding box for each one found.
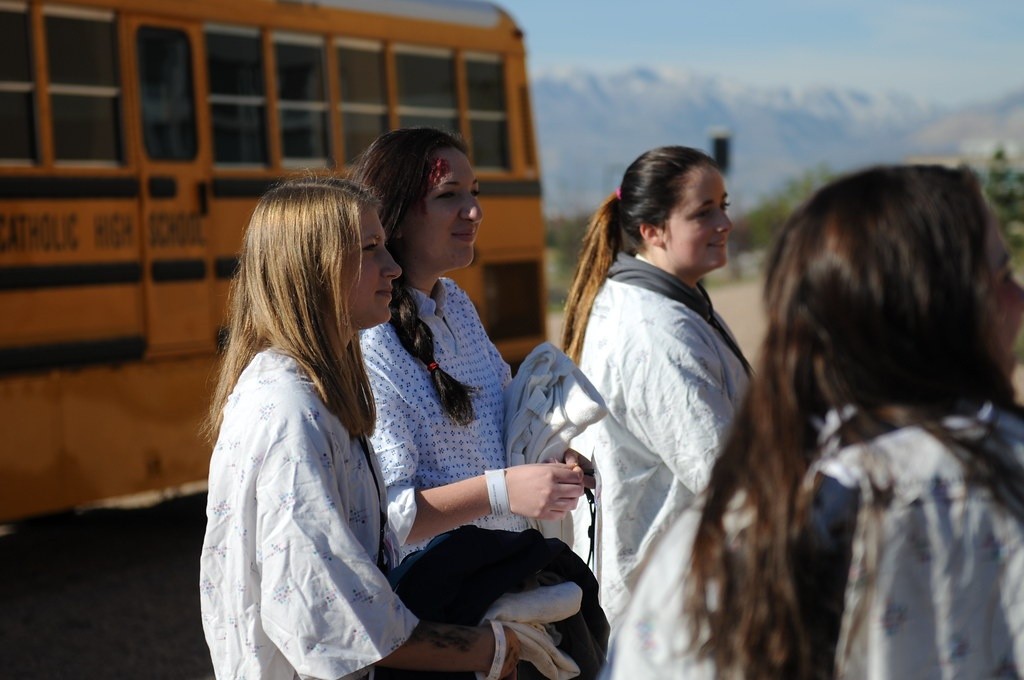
[0,0,554,522]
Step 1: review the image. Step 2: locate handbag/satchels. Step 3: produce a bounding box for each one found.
[365,525,610,680]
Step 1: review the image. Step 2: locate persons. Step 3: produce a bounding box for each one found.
[535,144,756,680]
[661,163,1024,680]
[196,174,520,680]
[338,125,597,565]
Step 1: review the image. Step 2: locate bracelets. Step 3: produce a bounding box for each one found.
[472,616,506,680]
[482,468,512,519]
